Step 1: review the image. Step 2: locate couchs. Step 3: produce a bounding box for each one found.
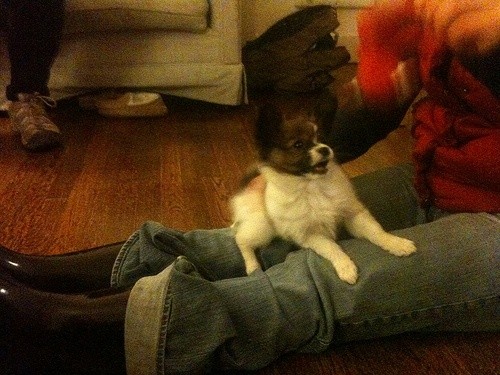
[0,0,248,111]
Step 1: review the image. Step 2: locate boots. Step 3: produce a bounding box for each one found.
[0,281,132,374]
[0,241,129,292]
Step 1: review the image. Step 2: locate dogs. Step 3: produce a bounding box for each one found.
[231,91,416,285]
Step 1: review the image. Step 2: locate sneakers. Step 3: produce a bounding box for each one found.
[8,95,68,152]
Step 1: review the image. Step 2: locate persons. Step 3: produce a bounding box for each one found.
[0,0,66,151]
[0,0,500,375]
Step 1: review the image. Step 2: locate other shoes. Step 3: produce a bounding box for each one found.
[78,92,167,119]
